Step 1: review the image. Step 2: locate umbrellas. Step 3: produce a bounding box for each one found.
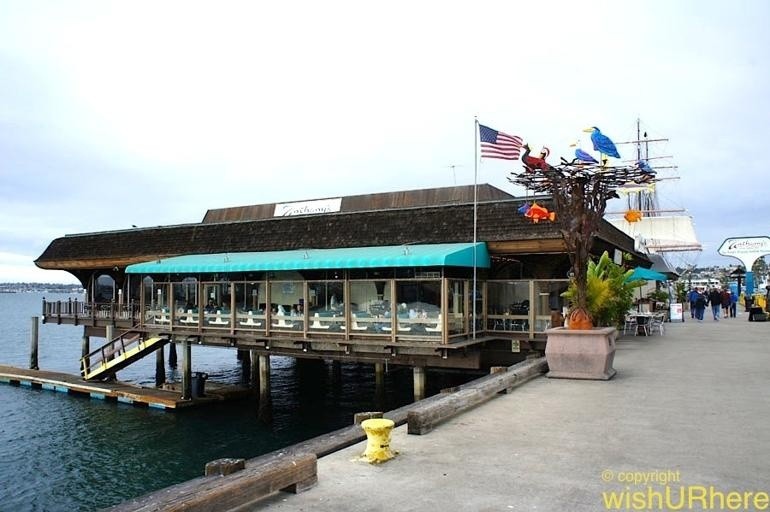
[623,265,667,311]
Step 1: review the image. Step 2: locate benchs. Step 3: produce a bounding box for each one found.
[407,356,549,435]
[133,453,317,512]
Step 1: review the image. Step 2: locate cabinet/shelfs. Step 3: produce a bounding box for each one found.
[670,302,683,323]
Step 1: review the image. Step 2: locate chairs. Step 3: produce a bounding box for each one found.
[145,301,531,335]
[623,308,667,337]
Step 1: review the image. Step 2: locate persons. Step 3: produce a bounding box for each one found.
[184,297,230,314]
[687,287,738,321]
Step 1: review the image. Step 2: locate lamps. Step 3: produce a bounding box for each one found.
[400,239,426,256]
[303,248,311,259]
[223,252,230,263]
[154,254,161,263]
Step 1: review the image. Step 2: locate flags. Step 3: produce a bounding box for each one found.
[479,124,523,160]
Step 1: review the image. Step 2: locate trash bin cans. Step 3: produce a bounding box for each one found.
[744,296,755,312]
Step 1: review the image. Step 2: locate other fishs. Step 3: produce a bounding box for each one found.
[623,209,643,225]
[524,202,556,224]
[615,182,656,197]
[518,204,530,213]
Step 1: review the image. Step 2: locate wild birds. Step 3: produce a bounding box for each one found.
[581,126,621,158]
[568,139,599,164]
[634,160,658,174]
[521,142,565,180]
